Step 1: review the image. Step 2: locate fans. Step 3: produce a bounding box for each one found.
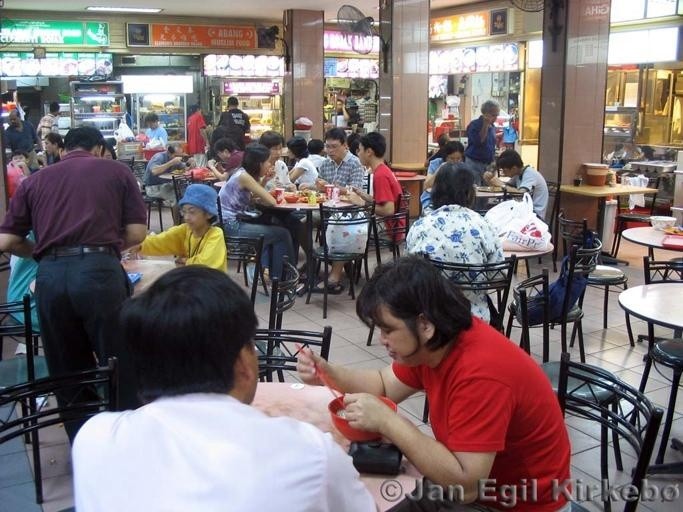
[337,4,388,73]
[257,25,290,72]
[511,0,562,53]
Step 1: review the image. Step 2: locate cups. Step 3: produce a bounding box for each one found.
[358,121,364,128]
[324,185,334,199]
[275,189,285,204]
[352,124,357,133]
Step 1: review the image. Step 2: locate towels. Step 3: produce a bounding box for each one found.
[628,174,649,211]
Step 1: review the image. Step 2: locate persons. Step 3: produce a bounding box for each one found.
[36,102,60,149]
[218,143,294,288]
[0,126,147,446]
[7,230,40,332]
[347,133,361,156]
[286,136,318,184]
[309,132,406,295]
[403,162,508,324]
[297,257,571,512]
[259,130,313,266]
[331,99,350,128]
[5,110,38,164]
[102,141,117,160]
[143,143,188,226]
[483,149,548,223]
[206,138,244,181]
[44,132,64,166]
[464,100,500,211]
[424,133,451,167]
[129,184,227,275]
[10,149,34,176]
[217,96,251,133]
[186,104,207,155]
[420,141,464,215]
[144,113,168,147]
[71,265,377,511]
[308,138,326,172]
[298,128,364,195]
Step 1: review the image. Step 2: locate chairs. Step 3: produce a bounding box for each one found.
[355,184,412,285]
[0,248,43,358]
[630,256,683,466]
[0,294,52,505]
[222,233,264,307]
[252,256,300,382]
[558,207,635,347]
[129,155,163,233]
[514,179,560,277]
[371,207,410,267]
[504,185,535,201]
[0,356,120,512]
[172,176,214,227]
[420,251,516,423]
[305,199,370,318]
[252,325,333,385]
[513,266,625,512]
[505,231,603,364]
[216,195,269,296]
[557,353,662,511]
[610,173,660,264]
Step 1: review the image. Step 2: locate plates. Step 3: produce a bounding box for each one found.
[662,235,683,250]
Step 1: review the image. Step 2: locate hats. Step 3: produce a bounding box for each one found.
[178,183,219,223]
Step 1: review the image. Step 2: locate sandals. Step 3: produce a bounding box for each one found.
[308,281,342,295]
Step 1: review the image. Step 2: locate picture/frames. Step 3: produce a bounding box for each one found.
[490,8,508,35]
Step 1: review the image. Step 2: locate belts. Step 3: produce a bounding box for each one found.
[43,245,117,258]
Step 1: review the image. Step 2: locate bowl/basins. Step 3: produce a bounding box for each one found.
[328,396,398,444]
[284,196,299,203]
[144,149,165,160]
[650,216,677,231]
[630,160,677,173]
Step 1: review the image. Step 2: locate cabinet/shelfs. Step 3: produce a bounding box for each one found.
[130,92,187,142]
[604,106,643,153]
[70,80,124,118]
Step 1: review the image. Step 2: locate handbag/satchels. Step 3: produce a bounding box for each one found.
[516,257,589,326]
